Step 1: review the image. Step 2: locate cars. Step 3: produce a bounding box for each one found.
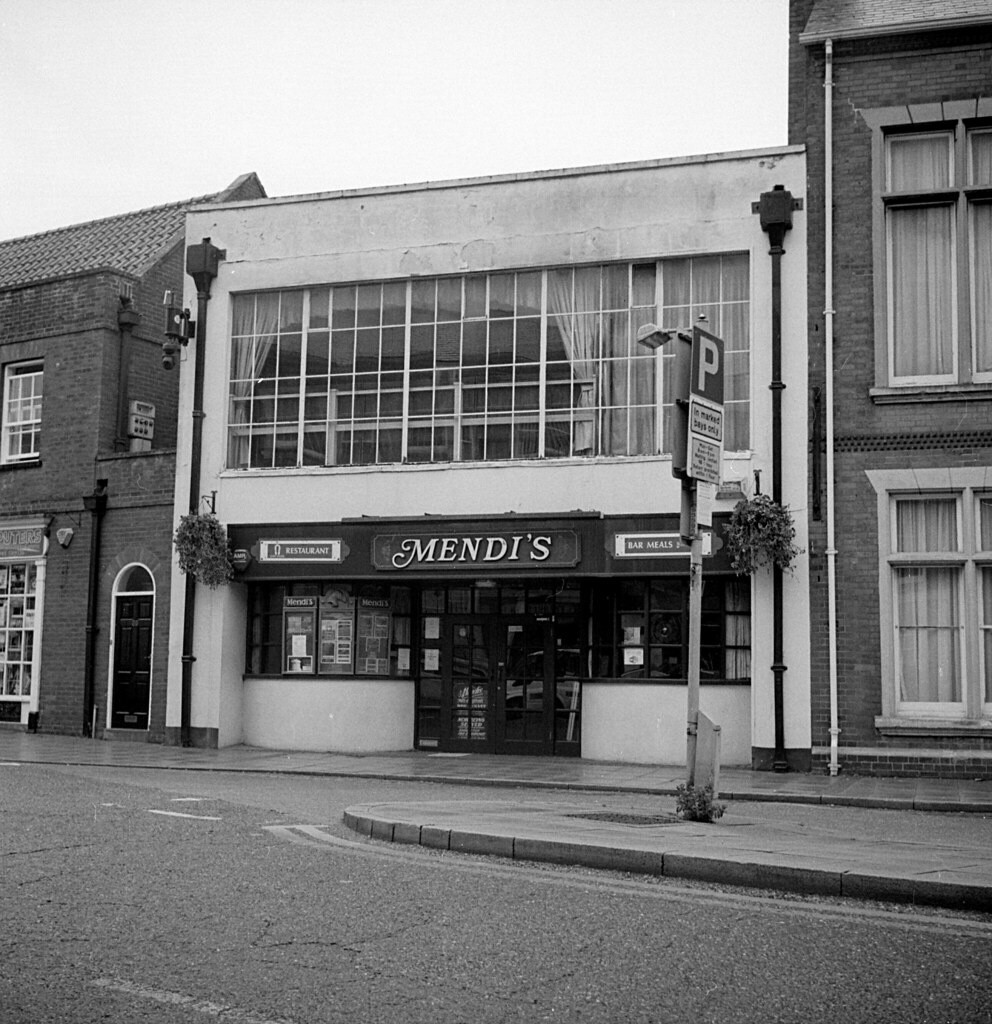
[427,646,585,719]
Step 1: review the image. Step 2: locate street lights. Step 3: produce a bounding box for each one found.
[638,313,704,781]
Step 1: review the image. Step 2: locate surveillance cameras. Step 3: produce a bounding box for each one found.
[162,354,177,370]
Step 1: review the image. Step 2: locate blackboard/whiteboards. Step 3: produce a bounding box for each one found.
[452,683,490,743]
